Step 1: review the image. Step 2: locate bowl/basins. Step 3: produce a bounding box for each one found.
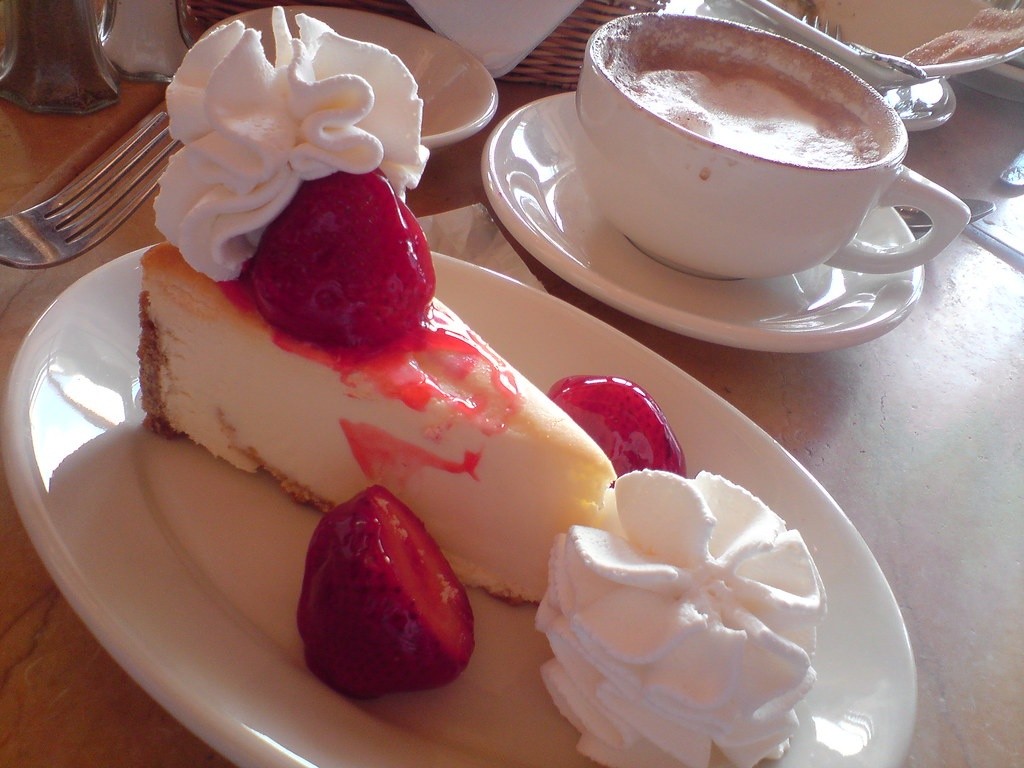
[737,0,1024,85]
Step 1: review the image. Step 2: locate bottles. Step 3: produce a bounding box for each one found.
[0,0,122,117]
[96,0,194,83]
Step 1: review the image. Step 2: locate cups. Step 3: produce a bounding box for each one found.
[575,12,973,281]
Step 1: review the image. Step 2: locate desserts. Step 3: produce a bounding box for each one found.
[536,467,830,765]
[136,15,614,599]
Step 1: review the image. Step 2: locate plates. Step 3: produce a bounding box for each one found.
[0,242,920,768]
[480,91,926,352]
[662,0,958,131]
[197,5,500,149]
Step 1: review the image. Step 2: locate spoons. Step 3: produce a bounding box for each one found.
[892,198,997,230]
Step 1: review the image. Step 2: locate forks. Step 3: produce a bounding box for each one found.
[798,13,928,81]
[0,112,188,268]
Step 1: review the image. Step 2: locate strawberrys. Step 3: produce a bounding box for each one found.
[298,489,471,698]
[552,375,685,478]
